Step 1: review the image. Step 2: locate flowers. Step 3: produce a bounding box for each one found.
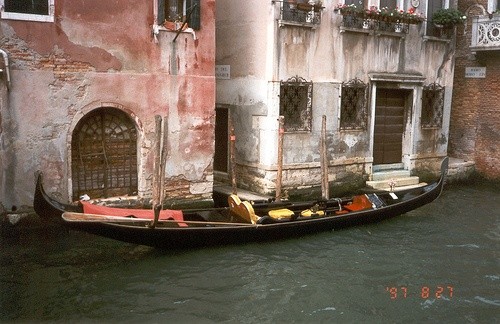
[333,2,425,33]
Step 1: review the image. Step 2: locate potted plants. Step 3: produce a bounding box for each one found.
[430,7,465,28]
[287,0,323,13]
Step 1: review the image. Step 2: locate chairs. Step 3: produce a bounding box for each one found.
[227,194,263,224]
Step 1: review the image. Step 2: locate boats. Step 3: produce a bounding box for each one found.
[33,108,449,249]
